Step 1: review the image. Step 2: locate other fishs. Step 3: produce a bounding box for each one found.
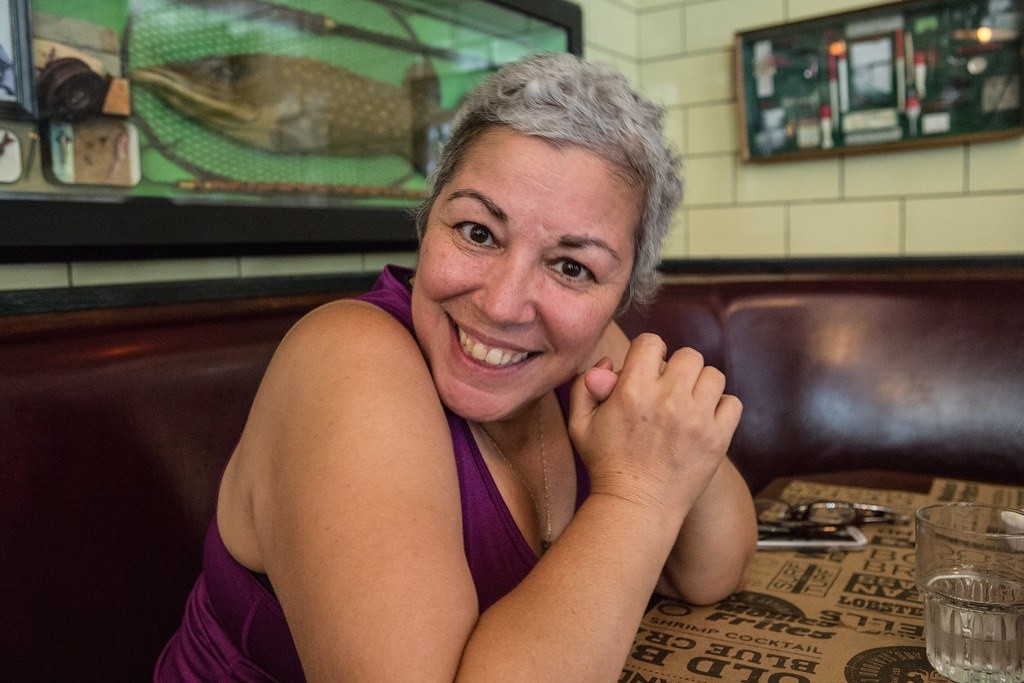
[128,52,455,180]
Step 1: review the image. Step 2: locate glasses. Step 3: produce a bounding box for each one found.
[754,496,857,529]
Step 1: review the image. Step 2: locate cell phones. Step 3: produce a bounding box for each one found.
[755,525,868,552]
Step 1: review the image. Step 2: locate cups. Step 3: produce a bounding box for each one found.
[914,501,1024,683]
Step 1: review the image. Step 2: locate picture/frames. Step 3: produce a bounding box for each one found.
[735,1,1023,165]
[3,0,586,269]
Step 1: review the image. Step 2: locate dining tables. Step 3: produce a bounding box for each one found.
[611,469,1022,683]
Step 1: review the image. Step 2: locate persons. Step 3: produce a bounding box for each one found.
[147,48,760,682]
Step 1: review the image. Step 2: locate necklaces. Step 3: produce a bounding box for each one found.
[472,396,552,557]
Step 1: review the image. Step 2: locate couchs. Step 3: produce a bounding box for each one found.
[0,253,1024,681]
